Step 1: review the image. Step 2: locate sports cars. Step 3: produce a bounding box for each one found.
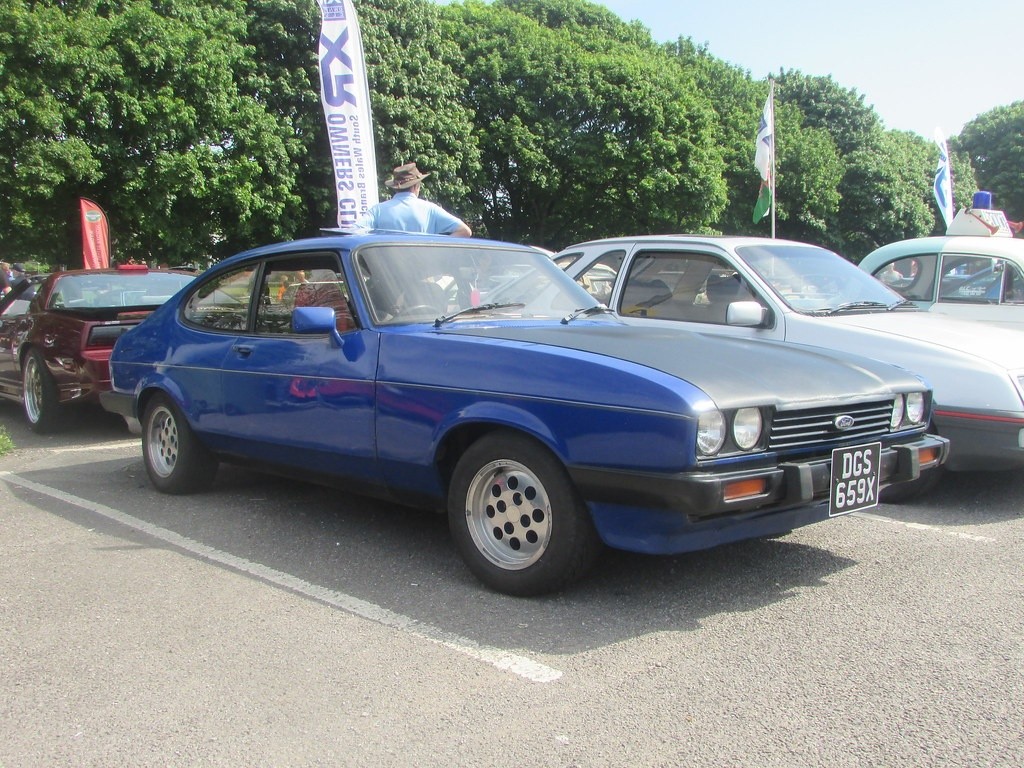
[101,227,953,599]
[0,263,245,434]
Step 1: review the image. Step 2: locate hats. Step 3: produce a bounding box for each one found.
[384,163,431,188]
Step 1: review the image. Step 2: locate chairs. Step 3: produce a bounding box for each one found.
[701,273,744,321]
[403,282,449,318]
[278,284,354,335]
[624,280,682,320]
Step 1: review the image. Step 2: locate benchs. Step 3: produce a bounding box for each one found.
[196,303,290,333]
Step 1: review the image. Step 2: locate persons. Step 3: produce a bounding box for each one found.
[881,262,902,285]
[351,163,474,239]
[0,261,28,299]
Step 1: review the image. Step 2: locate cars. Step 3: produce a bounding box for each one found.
[421,242,618,326]
[473,233,1024,505]
[841,235,1023,333]
